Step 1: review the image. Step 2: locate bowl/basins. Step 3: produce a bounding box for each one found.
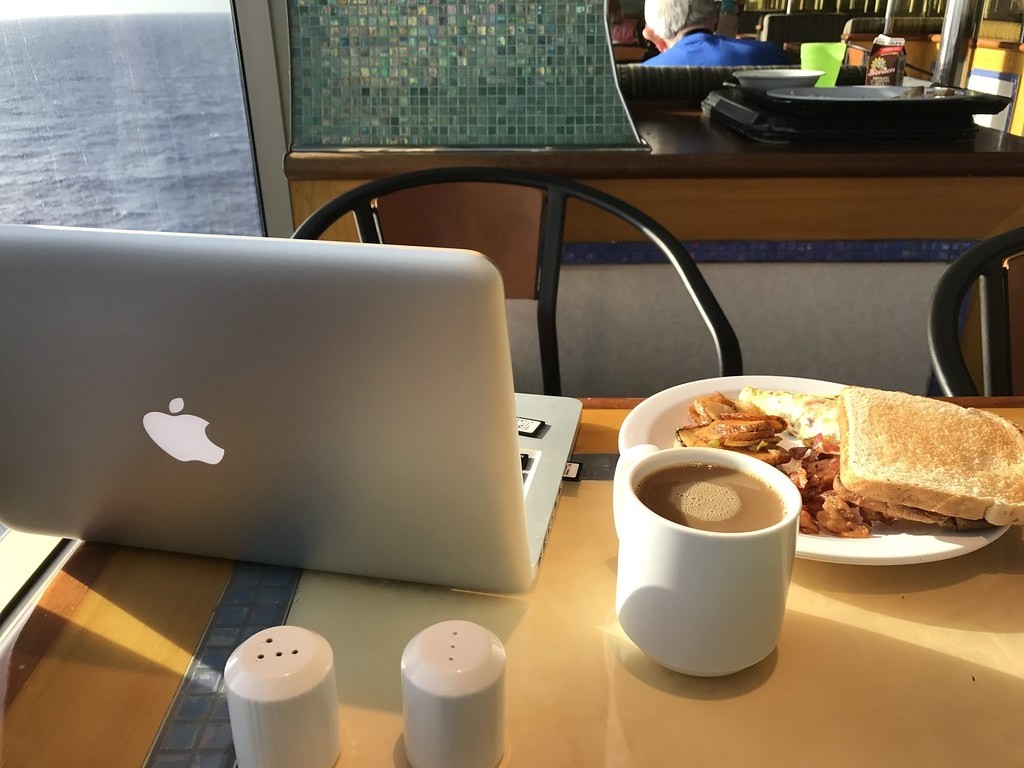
[733,68,825,100]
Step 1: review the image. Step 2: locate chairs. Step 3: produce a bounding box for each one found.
[286,166,742,395]
[926,225,1024,396]
[756,11,855,42]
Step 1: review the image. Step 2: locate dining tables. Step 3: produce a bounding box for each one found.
[0,396,1024,768]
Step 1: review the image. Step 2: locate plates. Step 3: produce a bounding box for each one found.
[613,375,1017,566]
[767,85,971,100]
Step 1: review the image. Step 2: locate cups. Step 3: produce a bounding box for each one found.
[610,443,804,678]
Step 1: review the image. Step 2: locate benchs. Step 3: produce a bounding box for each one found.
[922,20,1024,137]
[615,63,868,115]
[840,15,943,80]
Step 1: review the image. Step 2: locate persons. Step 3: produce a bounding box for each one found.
[640,0,794,67]
[606,0,638,43]
[716,0,747,39]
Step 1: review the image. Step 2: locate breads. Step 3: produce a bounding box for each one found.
[833,385,1024,528]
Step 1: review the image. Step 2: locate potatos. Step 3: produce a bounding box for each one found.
[674,391,792,466]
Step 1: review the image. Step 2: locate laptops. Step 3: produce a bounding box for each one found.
[0,223,583,596]
